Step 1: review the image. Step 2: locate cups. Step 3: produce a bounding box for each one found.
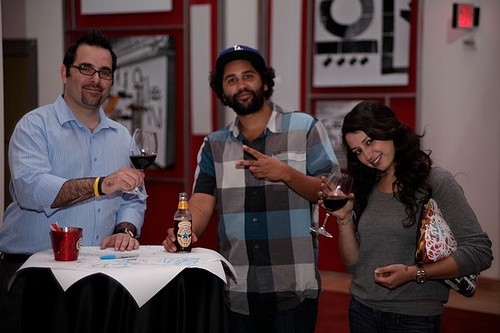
[49,228,83,261]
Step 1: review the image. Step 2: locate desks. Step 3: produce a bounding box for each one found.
[0,243,238,333]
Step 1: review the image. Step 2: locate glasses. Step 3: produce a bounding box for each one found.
[67,64,114,81]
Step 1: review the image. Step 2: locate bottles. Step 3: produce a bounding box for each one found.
[173,193,192,253]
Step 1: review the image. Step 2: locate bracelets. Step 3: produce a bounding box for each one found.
[337,212,352,225]
[94,177,105,196]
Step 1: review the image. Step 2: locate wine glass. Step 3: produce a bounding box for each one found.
[123,128,158,196]
[309,170,353,237]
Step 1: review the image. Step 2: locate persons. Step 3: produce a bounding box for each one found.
[318,100,493,333]
[0,40,147,297]
[163,46,341,333]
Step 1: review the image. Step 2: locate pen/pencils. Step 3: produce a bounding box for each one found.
[100,254,140,260]
[49,221,75,232]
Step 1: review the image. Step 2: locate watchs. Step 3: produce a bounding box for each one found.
[113,227,134,237]
[416,264,427,283]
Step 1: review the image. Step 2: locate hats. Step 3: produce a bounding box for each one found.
[215,45,265,72]
